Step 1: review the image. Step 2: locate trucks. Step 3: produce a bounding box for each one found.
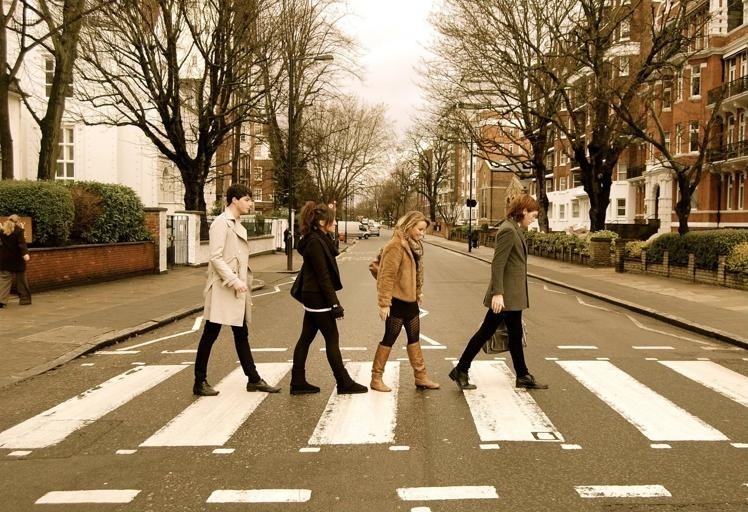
[335,220,372,240]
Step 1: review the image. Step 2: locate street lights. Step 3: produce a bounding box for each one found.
[284,53,338,271]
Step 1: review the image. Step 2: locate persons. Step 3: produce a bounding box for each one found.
[0,212,33,309]
[448,196,551,390]
[193,183,283,397]
[371,210,441,393]
[470,230,479,248]
[284,228,289,255]
[289,202,369,395]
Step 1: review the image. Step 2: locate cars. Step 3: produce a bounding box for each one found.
[359,215,382,237]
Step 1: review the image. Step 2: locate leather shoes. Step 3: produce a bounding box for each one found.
[448,367,477,389]
[246,378,281,393]
[193,380,220,396]
[515,374,549,389]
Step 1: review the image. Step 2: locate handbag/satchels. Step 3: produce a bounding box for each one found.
[480,318,528,355]
[369,247,384,280]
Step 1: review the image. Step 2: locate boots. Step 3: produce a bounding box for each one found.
[333,368,368,394]
[407,340,441,390]
[289,368,321,396]
[369,341,392,392]
